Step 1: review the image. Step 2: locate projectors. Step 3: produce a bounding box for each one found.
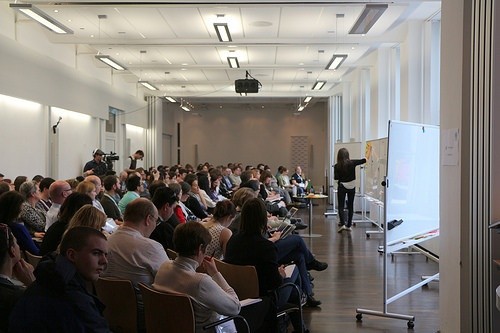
[235,79,258,93]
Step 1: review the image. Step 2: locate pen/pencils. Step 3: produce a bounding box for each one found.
[208,251,215,261]
[283,261,294,269]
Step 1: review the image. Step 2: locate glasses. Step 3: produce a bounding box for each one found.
[185,192,190,196]
[64,187,72,193]
[169,192,176,199]
[0,223,9,244]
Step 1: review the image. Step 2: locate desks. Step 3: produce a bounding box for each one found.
[292,193,329,259]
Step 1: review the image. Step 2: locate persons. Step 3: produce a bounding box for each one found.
[100,198,172,314]
[334,142,372,233]
[152,221,241,333]
[0,148,329,333]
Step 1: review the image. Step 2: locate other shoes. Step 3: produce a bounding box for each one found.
[307,295,321,306]
[306,259,328,271]
[295,223,307,230]
[346,226,352,230]
[338,225,345,232]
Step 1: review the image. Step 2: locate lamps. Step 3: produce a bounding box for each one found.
[9,3,74,35]
[297,85,306,112]
[164,90,178,103]
[309,50,329,92]
[227,50,240,69]
[325,14,352,71]
[138,69,159,92]
[212,15,233,43]
[95,46,129,71]
[303,72,313,103]
[182,100,191,112]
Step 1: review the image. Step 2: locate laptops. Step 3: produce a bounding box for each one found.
[278,206,298,223]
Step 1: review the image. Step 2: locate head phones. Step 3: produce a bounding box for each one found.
[92,148,98,157]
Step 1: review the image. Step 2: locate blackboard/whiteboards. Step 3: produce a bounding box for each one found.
[364,137,388,203]
[384,120,440,253]
[333,142,362,194]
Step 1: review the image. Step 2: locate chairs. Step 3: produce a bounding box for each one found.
[92,275,140,333]
[138,280,251,333]
[166,248,304,333]
[25,249,42,268]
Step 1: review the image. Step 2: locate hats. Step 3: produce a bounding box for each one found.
[93,149,105,156]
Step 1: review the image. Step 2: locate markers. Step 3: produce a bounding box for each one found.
[415,236,424,240]
